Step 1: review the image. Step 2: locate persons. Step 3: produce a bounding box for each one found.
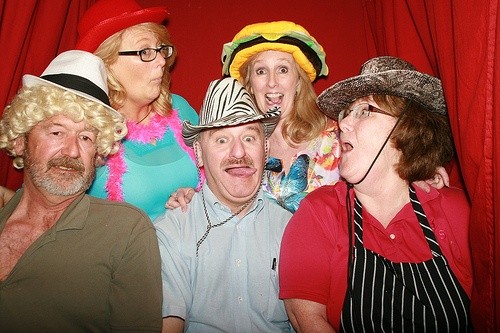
[0,0,207,222]
[0,49,164,333]
[149,77,301,333]
[278,56,476,333]
[163,20,450,215]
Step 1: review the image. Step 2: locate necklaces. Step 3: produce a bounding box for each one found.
[136,107,153,124]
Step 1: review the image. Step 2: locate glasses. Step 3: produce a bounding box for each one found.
[338,103,404,124]
[117,45,174,62]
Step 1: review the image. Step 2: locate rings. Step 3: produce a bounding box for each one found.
[170,192,178,201]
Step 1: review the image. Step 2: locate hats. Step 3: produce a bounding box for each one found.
[181,77,282,149]
[22,50,122,118]
[221,21,329,83]
[76,0,170,54]
[316,56,447,121]
